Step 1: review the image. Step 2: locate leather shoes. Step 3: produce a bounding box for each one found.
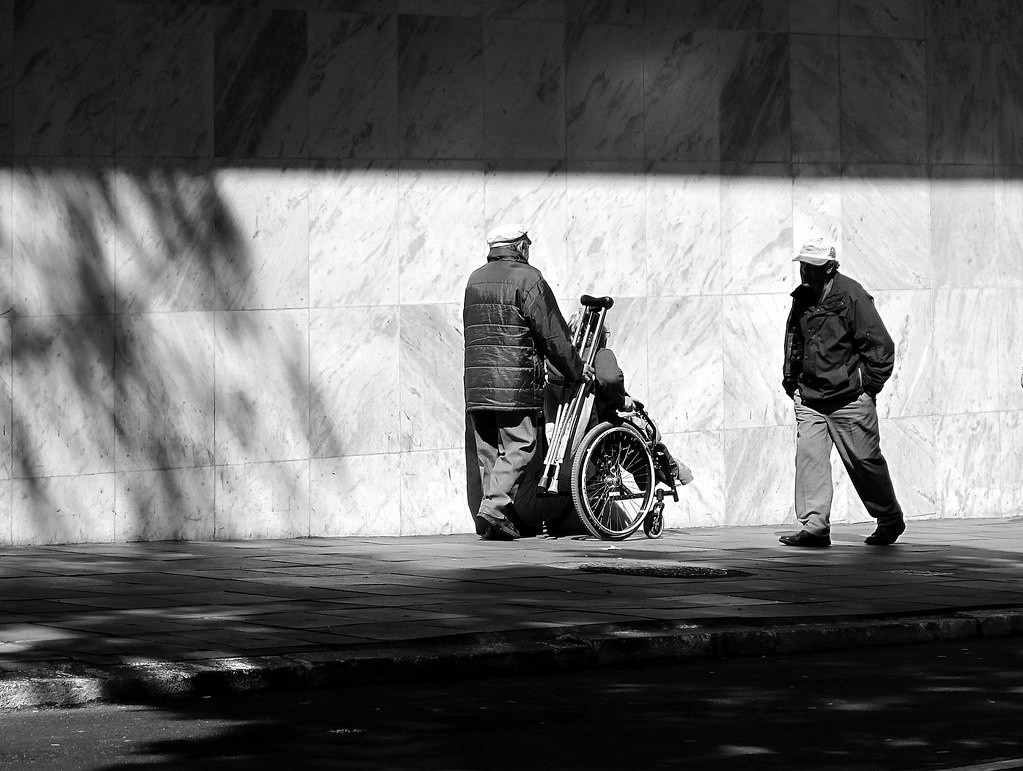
[779,531,831,547]
[864,522,905,546]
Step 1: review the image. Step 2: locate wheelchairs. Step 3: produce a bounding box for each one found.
[570,392,679,541]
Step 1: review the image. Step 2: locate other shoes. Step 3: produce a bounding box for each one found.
[476,513,519,538]
[677,464,693,486]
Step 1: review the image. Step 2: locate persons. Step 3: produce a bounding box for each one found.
[541,307,695,490]
[462,221,594,538]
[778,237,906,548]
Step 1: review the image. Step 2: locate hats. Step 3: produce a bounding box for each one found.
[791,239,837,267]
[486,224,532,250]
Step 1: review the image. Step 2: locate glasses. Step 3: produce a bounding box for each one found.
[603,330,611,338]
